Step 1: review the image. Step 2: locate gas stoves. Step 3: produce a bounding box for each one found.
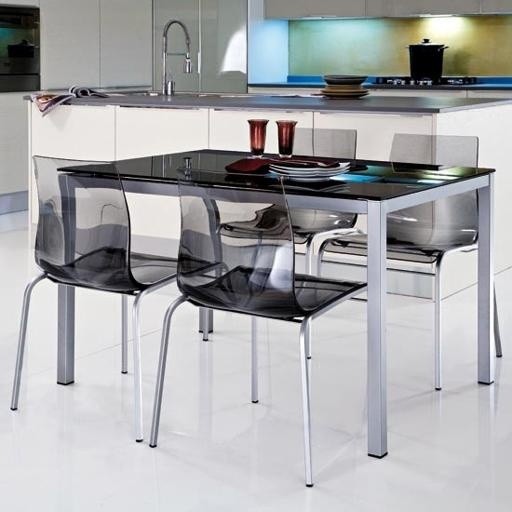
[369,76,480,88]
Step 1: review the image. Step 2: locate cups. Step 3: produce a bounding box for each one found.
[247,116,298,160]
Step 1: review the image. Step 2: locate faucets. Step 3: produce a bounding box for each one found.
[160,17,193,96]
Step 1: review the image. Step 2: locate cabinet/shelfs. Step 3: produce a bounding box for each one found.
[205,111,437,278]
[263,0,512,20]
[39,0,248,95]
[28,103,208,235]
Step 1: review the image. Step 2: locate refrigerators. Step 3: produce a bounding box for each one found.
[1,1,39,215]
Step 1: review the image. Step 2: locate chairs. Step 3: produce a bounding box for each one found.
[149,167,373,487]
[11,155,198,445]
[304,132,503,391]
[206,129,364,357]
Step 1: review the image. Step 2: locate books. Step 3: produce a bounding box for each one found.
[31,86,109,117]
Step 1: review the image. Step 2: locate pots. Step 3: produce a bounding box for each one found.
[409,36,446,79]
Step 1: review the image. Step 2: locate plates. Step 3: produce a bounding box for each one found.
[266,156,352,183]
[319,74,369,100]
